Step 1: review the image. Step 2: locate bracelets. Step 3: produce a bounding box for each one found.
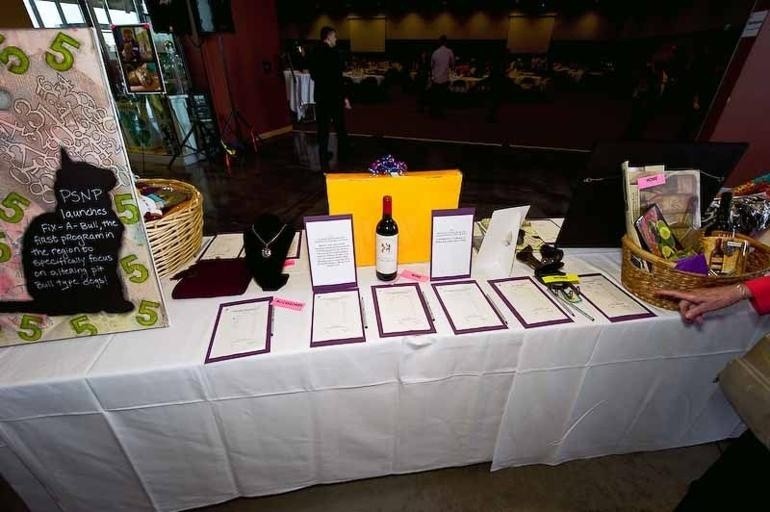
[736,282,746,298]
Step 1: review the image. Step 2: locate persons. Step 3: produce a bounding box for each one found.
[430,34,456,122]
[655,274,770,328]
[306,26,354,174]
[112,24,168,97]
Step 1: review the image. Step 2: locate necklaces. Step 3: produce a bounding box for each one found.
[252,219,287,259]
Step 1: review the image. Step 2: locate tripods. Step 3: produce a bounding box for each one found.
[218,32,265,152]
[169,34,223,169]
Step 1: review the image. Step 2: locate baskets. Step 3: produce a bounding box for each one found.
[620,230,770,311]
[136,178,205,279]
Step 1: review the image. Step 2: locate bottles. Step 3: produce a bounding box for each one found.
[705,191,735,237]
[710,238,725,273]
[375,195,399,282]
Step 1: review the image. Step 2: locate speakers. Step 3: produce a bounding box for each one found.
[144,0,192,34]
[189,0,235,36]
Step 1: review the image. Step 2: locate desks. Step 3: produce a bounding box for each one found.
[0,232,770,510]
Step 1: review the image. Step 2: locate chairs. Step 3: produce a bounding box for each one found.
[340,59,590,108]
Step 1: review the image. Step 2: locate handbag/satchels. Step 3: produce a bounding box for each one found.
[718,335,770,448]
